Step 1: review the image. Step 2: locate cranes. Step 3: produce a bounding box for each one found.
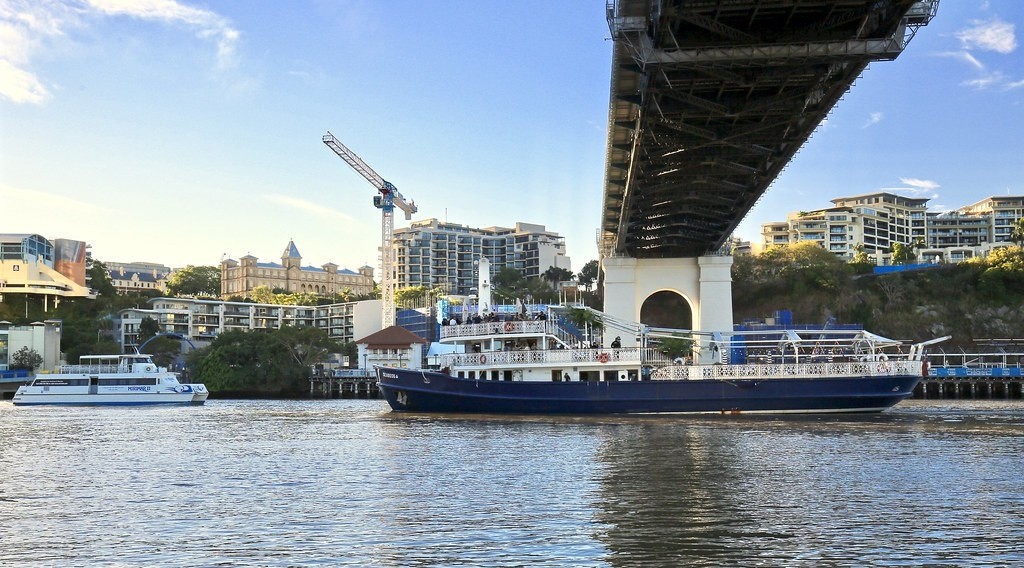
[319,130,419,329]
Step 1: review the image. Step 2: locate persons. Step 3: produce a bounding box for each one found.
[504,343,512,351]
[534,311,546,329]
[611,338,620,348]
[514,342,537,350]
[505,311,523,321]
[564,372,571,381]
[441,311,499,326]
[592,342,598,348]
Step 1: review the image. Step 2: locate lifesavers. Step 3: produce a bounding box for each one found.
[504,322,516,332]
[480,355,487,364]
[876,353,888,362]
[598,353,608,363]
[673,357,686,366]
[860,356,869,362]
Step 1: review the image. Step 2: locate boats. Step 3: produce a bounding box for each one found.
[9,350,212,408]
[368,292,932,413]
